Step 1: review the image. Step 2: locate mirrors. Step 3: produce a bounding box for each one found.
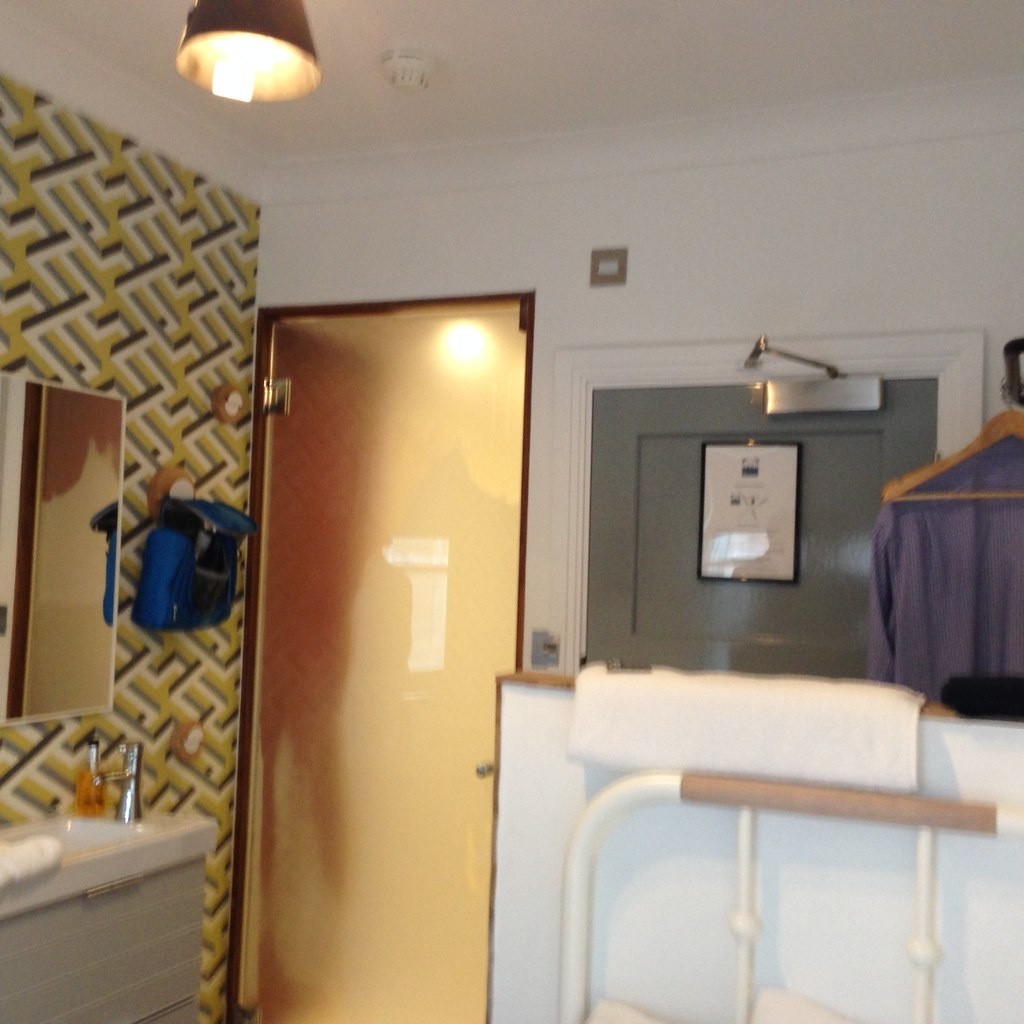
[24,384,124,713]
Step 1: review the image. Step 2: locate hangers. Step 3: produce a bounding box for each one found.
[881,374,1024,505]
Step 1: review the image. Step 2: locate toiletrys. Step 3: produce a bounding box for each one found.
[76,742,106,817]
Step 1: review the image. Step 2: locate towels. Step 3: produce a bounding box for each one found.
[0,834,62,890]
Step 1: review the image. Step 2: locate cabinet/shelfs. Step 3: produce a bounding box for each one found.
[0,853,203,1023]
[0,370,127,727]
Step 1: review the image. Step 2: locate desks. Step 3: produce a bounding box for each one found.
[482,670,1024,1023]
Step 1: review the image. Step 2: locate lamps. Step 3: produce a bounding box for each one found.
[172,1,322,104]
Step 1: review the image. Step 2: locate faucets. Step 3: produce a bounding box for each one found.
[94,741,145,825]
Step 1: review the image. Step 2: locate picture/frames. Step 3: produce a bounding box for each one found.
[697,436,803,585]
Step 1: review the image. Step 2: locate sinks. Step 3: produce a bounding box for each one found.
[2,815,157,864]
